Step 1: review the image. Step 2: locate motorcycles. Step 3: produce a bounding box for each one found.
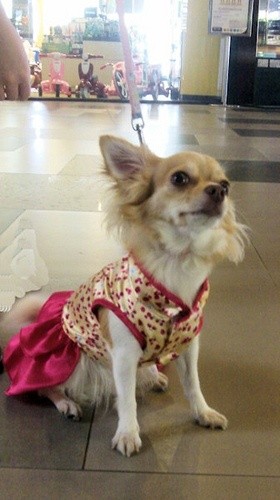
[100,60,150,102]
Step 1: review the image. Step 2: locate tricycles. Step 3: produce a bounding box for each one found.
[142,63,169,100]
[75,54,108,99]
[36,53,78,97]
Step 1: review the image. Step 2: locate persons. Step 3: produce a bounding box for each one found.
[0,1,32,101]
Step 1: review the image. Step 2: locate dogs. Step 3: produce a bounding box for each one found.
[0,134,254,459]
